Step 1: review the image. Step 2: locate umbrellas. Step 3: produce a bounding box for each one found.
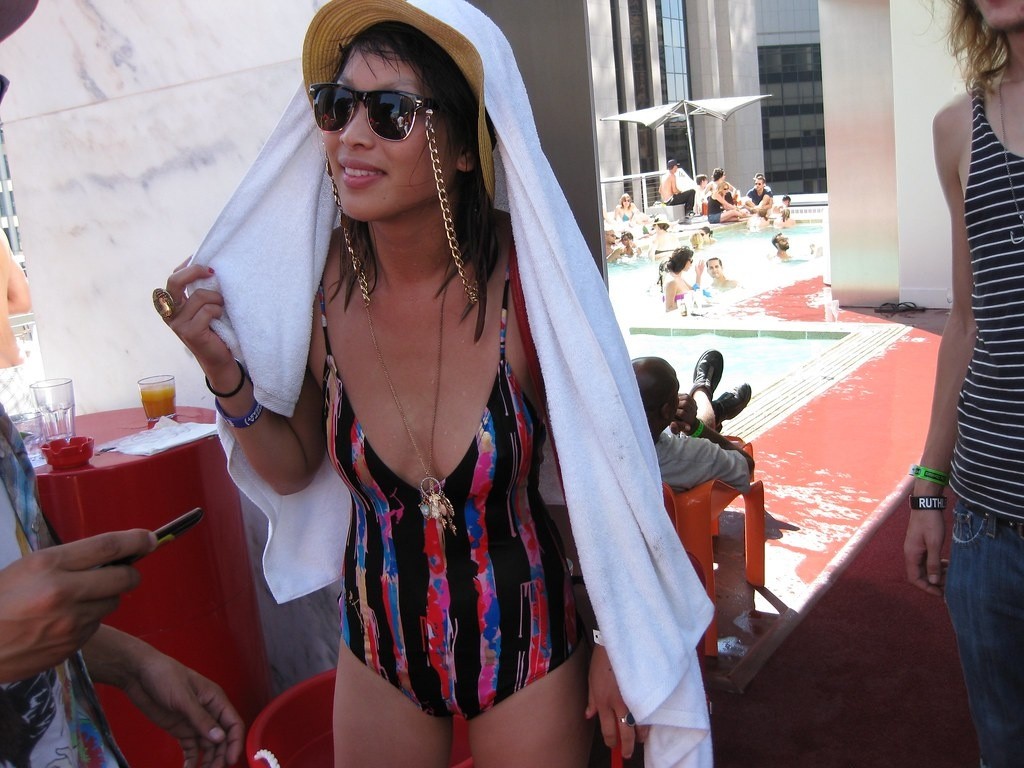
[599,94,773,184]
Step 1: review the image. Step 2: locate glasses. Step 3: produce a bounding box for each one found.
[708,265,719,270]
[777,238,787,241]
[689,258,693,265]
[624,199,630,202]
[700,233,706,236]
[608,234,616,237]
[307,81,455,142]
[755,182,761,185]
[721,188,728,191]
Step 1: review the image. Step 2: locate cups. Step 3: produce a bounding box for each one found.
[137,374,177,422]
[677,289,703,311]
[29,378,76,441]
[8,411,51,469]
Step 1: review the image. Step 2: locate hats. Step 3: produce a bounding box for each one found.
[669,159,681,166]
[302,0,495,206]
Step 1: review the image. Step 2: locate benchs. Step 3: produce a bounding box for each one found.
[646,204,685,224]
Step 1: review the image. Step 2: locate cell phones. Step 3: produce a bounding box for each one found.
[103,508,205,571]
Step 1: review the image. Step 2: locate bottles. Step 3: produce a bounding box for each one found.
[680,303,687,316]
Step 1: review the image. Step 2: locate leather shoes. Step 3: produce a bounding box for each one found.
[687,350,724,400]
[712,384,752,434]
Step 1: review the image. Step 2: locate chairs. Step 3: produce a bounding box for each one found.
[659,435,765,656]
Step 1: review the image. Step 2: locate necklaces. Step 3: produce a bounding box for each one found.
[999,80,1024,223]
[360,270,457,535]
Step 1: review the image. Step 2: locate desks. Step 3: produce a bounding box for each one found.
[14,407,278,768]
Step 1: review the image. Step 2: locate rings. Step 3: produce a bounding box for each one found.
[152,288,182,319]
[619,710,635,726]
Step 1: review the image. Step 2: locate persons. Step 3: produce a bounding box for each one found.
[603,161,797,313]
[904,0,1024,768]
[152,0,714,768]
[0,404,244,768]
[0,230,31,370]
[631,349,757,496]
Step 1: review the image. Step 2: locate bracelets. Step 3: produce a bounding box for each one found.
[688,419,704,437]
[591,631,605,648]
[205,359,246,397]
[215,395,263,428]
[908,494,947,510]
[910,465,949,487]
